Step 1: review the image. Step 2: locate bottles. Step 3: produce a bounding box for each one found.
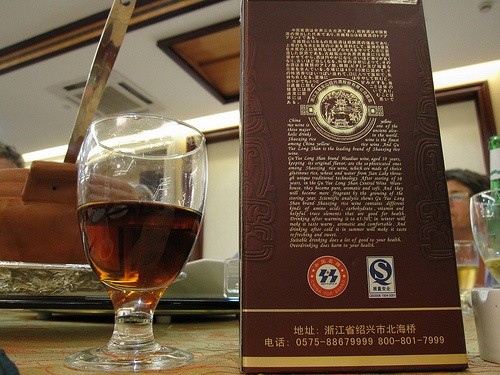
[487,136,500,251]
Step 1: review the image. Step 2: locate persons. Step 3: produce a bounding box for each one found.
[444,168,493,288]
[0,141,26,170]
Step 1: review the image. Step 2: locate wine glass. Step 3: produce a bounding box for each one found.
[453,240,478,313]
[470,190,500,283]
[65,114,207,371]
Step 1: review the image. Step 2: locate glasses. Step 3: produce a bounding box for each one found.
[450,191,468,202]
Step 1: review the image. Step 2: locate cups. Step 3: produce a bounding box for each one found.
[471,289,500,364]
